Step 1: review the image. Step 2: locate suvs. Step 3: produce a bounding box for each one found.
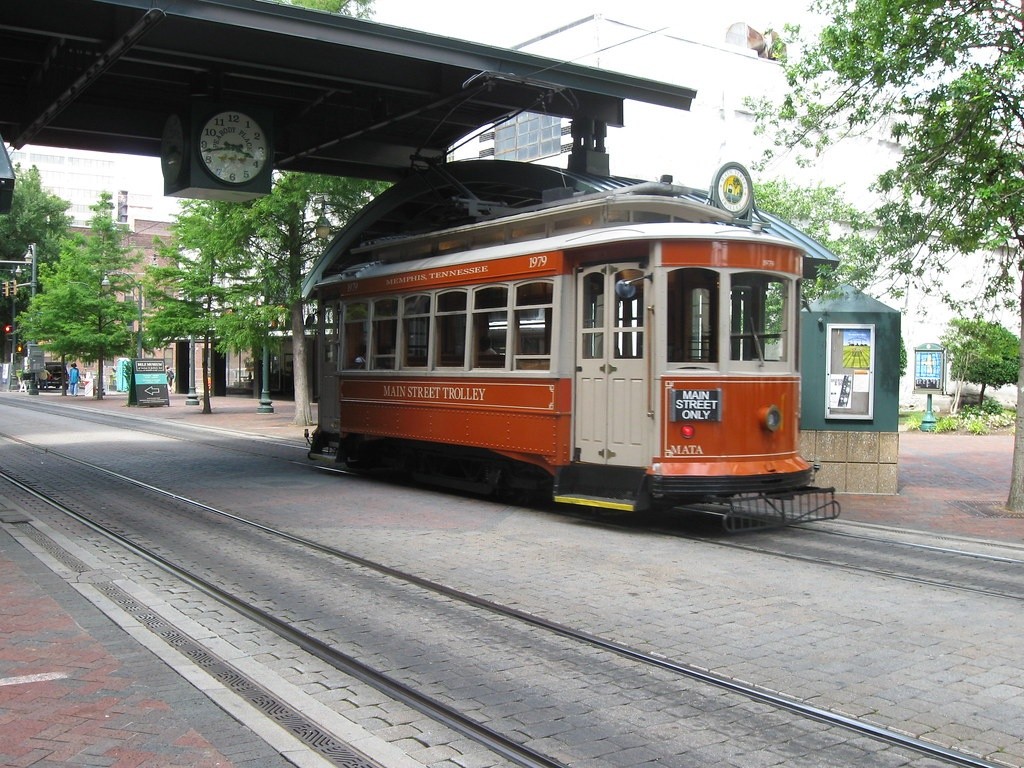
[40,362,70,390]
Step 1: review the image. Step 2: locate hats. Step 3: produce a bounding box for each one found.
[71,362,77,367]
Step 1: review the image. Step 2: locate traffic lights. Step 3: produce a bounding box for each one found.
[1,281,9,296]
[17,346,23,352]
[5,326,12,333]
[10,280,17,296]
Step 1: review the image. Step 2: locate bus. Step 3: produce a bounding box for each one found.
[304,155,841,533]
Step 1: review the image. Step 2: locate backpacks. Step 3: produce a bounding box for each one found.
[168,371,174,379]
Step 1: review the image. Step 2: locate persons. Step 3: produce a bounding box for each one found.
[165,365,173,393]
[40,370,51,392]
[478,336,498,356]
[69,362,81,397]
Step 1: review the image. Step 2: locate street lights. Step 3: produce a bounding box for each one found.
[16,242,37,396]
[101,272,142,358]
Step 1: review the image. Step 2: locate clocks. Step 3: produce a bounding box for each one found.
[161,111,188,185]
[198,110,269,187]
